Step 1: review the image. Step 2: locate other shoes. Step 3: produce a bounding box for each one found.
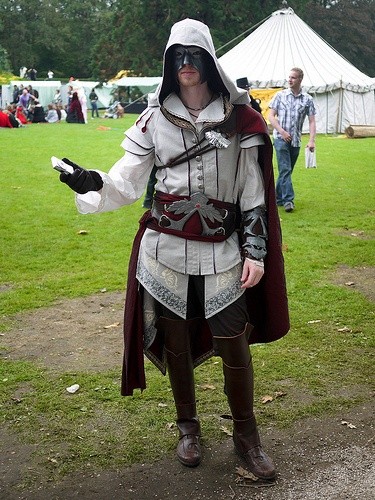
[284,202,293,211]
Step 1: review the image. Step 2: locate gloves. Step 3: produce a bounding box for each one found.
[60,158,95,195]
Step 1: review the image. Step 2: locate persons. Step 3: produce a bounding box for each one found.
[27,67,37,81]
[267,68,316,212]
[59,17,289,481]
[89,88,99,118]
[20,65,27,78]
[129,94,146,104]
[237,77,261,113]
[48,70,54,79]
[64,77,87,124]
[0,84,68,128]
[110,93,124,118]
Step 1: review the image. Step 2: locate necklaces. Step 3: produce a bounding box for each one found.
[183,94,214,118]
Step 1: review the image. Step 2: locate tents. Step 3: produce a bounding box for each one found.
[216,8,375,134]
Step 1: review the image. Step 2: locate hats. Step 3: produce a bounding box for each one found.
[236,78,251,88]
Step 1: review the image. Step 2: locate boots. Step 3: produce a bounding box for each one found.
[154,315,203,468]
[213,323,275,480]
